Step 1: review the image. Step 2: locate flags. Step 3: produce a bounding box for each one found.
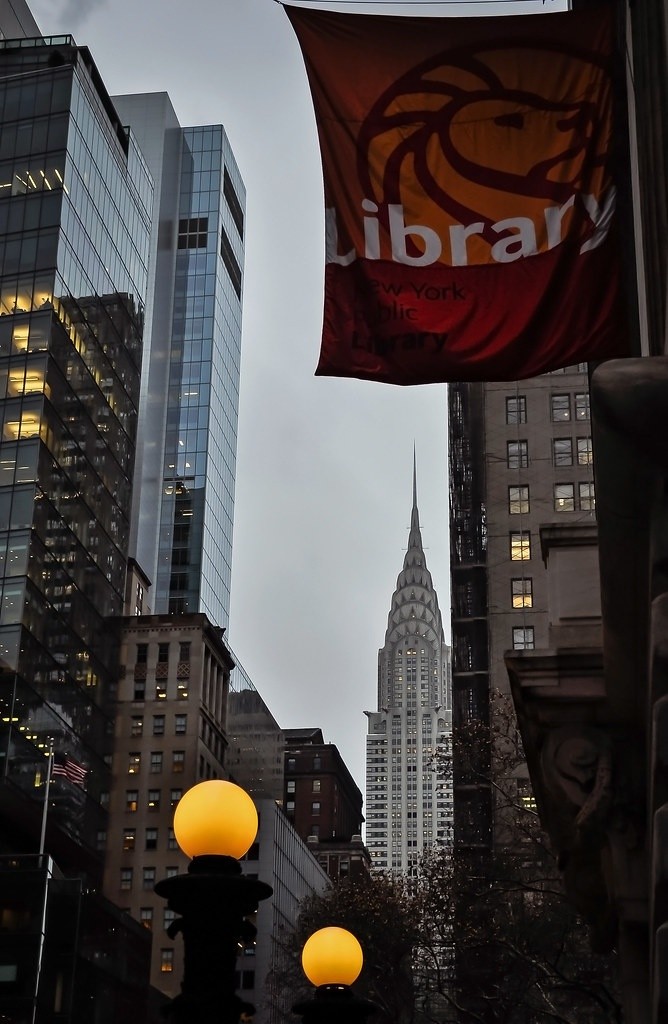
[288,1,643,386]
[51,752,88,785]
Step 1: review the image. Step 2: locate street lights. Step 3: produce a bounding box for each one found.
[292,926,384,1024]
[153,780,272,1024]
